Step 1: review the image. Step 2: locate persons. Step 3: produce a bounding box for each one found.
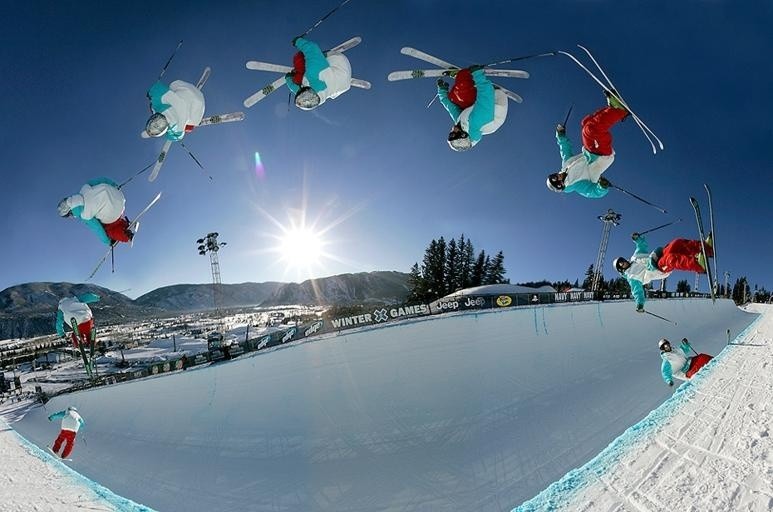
[56,293,104,348]
[48,407,84,459]
[285,36,352,111]
[612,232,714,313]
[546,90,628,199]
[145,80,205,141]
[57,176,133,247]
[658,338,714,386]
[437,65,508,152]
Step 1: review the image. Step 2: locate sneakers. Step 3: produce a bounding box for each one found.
[695,251,708,274]
[706,231,714,258]
[603,88,630,114]
[442,65,461,80]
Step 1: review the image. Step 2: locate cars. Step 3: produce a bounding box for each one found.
[91,356,113,368]
[133,355,166,366]
[34,362,44,372]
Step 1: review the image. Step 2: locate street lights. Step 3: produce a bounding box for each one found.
[194,230,229,348]
[589,205,625,294]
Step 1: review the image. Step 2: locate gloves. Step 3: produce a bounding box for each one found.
[556,124,567,134]
[469,65,485,73]
[291,36,303,48]
[598,177,611,189]
[636,304,645,313]
[668,380,675,386]
[436,79,450,90]
[632,232,640,241]
[682,337,689,345]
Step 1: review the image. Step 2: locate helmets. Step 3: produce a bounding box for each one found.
[447,135,472,152]
[68,406,77,411]
[546,174,564,193]
[56,197,72,217]
[145,113,169,137]
[295,88,320,111]
[659,338,671,350]
[613,256,624,273]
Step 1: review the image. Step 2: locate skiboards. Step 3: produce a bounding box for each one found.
[244,37,372,108]
[558,45,664,155]
[71,317,96,385]
[46,445,73,463]
[142,67,245,183]
[83,191,161,281]
[388,47,529,103]
[725,327,732,345]
[689,183,718,303]
